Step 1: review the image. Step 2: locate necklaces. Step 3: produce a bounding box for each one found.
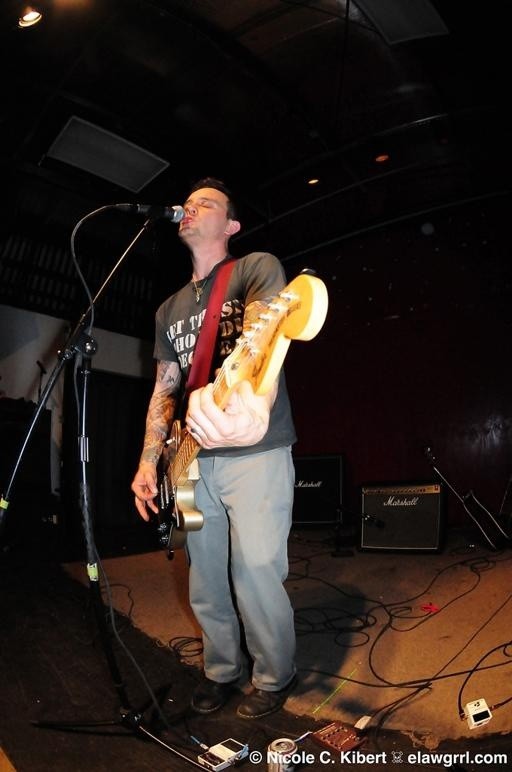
[191,267,212,304]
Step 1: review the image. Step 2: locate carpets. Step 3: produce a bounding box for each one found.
[61,528,512,754]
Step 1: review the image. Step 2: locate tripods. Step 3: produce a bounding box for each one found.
[0,223,212,772]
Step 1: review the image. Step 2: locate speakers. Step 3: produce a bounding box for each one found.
[292,455,343,525]
[61,368,161,562]
[358,481,446,554]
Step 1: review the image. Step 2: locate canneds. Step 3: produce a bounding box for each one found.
[267,737,298,772]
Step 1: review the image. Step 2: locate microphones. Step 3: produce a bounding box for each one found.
[116,204,184,223]
[368,517,387,528]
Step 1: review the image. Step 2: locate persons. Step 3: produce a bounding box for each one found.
[130,176,301,722]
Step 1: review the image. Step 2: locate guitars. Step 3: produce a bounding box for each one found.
[155,268,329,550]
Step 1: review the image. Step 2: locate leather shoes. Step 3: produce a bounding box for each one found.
[235,674,300,721]
[189,676,236,716]
